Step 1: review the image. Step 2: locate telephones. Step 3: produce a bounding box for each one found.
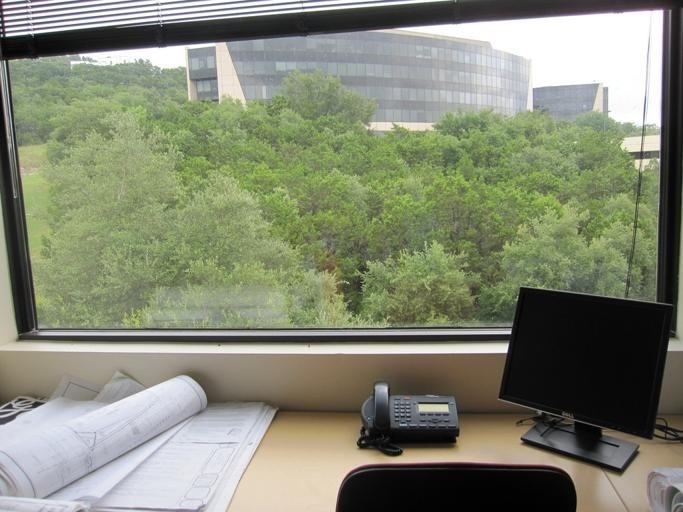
[356,381,459,441]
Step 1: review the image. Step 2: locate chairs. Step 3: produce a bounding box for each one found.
[336,464,577,512]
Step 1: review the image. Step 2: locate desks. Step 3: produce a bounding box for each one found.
[226,411,681,511]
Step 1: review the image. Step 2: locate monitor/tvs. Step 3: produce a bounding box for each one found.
[496,286,676,472]
